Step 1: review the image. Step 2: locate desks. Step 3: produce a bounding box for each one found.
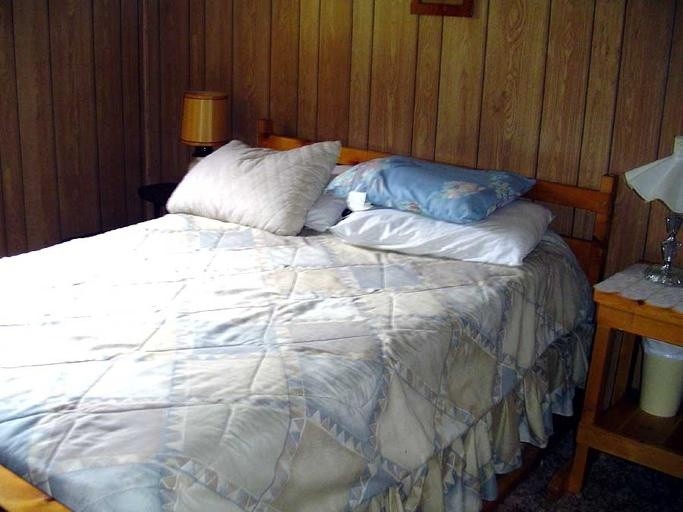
[565,261,683,494]
[137,183,176,219]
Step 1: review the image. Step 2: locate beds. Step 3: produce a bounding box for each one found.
[1,118,618,511]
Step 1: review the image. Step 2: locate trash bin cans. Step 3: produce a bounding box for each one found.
[639,337,683,417]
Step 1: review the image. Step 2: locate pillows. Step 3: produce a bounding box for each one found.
[166,138,341,236]
[322,153,538,225]
[303,164,354,233]
[327,200,556,268]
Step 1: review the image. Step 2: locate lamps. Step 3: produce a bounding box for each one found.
[181,89,228,171]
[619,135,683,287]
